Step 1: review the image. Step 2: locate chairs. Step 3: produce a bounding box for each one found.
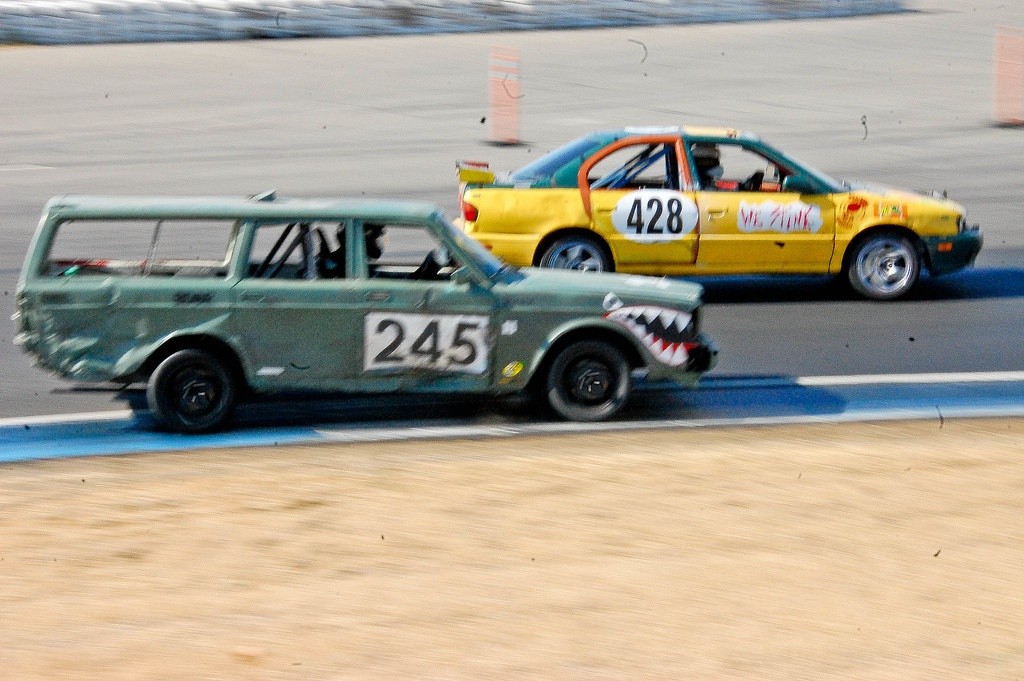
[314,226,335,277]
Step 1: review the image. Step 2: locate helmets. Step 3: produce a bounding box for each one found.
[691,147,722,188]
[336,221,386,260]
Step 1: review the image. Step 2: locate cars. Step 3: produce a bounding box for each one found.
[451,125,984,301]
[10,190,719,434]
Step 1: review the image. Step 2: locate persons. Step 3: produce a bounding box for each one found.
[319,223,452,279]
[692,146,751,191]
